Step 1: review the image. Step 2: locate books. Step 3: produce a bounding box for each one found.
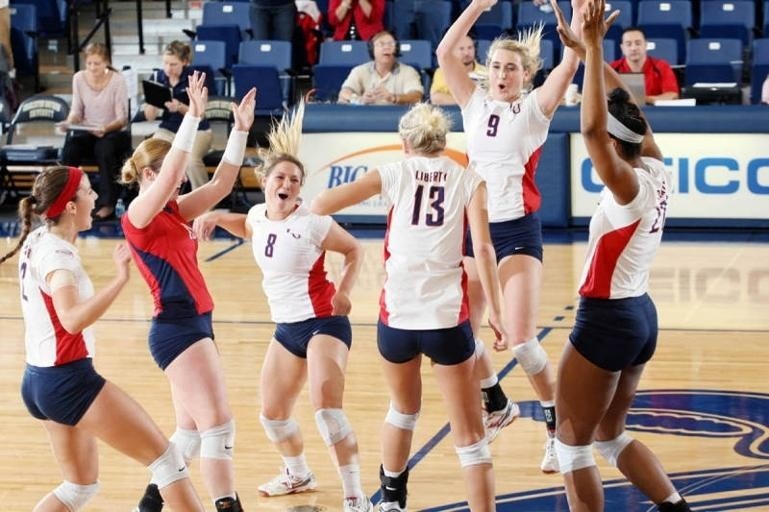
[141,78,172,111]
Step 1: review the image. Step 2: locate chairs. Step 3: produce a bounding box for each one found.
[3,0,74,209]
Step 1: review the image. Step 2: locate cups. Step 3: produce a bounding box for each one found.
[565,84,579,107]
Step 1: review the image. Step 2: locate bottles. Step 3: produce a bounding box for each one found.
[113,197,126,231]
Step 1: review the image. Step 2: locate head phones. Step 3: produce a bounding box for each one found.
[368,32,401,59]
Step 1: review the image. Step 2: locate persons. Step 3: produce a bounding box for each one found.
[429,0,591,476]
[127,41,213,214]
[191,93,372,512]
[113,69,260,512]
[429,34,492,106]
[0,164,210,512]
[605,28,681,104]
[58,41,137,226]
[247,0,384,79]
[546,0,692,511]
[335,30,426,107]
[311,98,512,511]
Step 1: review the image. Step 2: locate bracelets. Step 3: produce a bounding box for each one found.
[350,93,361,104]
[220,127,250,169]
[390,94,401,104]
[168,110,204,155]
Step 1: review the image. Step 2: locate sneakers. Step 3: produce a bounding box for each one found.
[342,492,374,512]
[378,499,408,512]
[483,397,521,445]
[258,467,316,498]
[540,428,561,474]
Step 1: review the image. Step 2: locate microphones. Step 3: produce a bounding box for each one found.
[392,52,400,58]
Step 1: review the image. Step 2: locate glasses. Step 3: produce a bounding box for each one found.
[374,39,397,48]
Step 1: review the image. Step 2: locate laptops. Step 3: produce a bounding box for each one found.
[620,72,646,104]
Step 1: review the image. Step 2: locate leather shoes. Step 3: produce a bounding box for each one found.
[92,205,116,222]
